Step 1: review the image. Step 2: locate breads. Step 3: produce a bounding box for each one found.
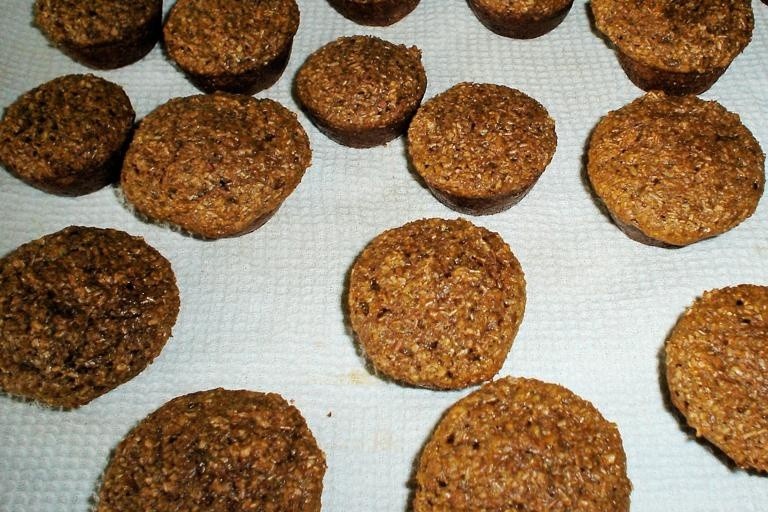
[0,226,181,407]
[406,374,632,511]
[404,80,558,218]
[1,0,426,240]
[587,0,755,96]
[95,387,326,512]
[665,284,767,475]
[467,0,574,39]
[342,218,528,390]
[588,91,765,251]
